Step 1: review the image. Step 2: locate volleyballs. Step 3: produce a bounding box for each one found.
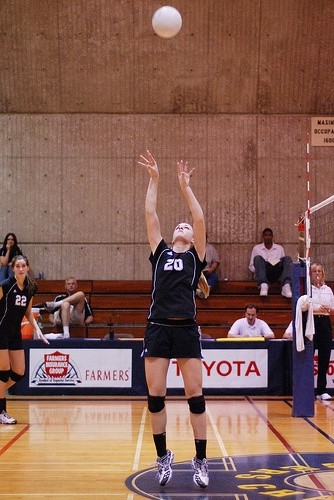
[150,6,182,38]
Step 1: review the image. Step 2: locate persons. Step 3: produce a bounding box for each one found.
[0,233,25,281]
[205,232,218,293]
[228,304,276,339]
[310,263,334,400]
[135,150,210,489]
[43,276,94,338]
[0,255,50,424]
[282,321,293,339]
[249,228,293,298]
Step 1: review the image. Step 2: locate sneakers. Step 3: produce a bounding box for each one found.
[43,301,55,310]
[259,283,268,295]
[317,393,332,400]
[280,284,292,298]
[0,411,17,425]
[155,450,174,487]
[191,456,209,488]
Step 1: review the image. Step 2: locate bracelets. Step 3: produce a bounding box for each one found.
[328,306,330,313]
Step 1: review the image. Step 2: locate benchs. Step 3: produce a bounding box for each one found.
[21,278,334,339]
